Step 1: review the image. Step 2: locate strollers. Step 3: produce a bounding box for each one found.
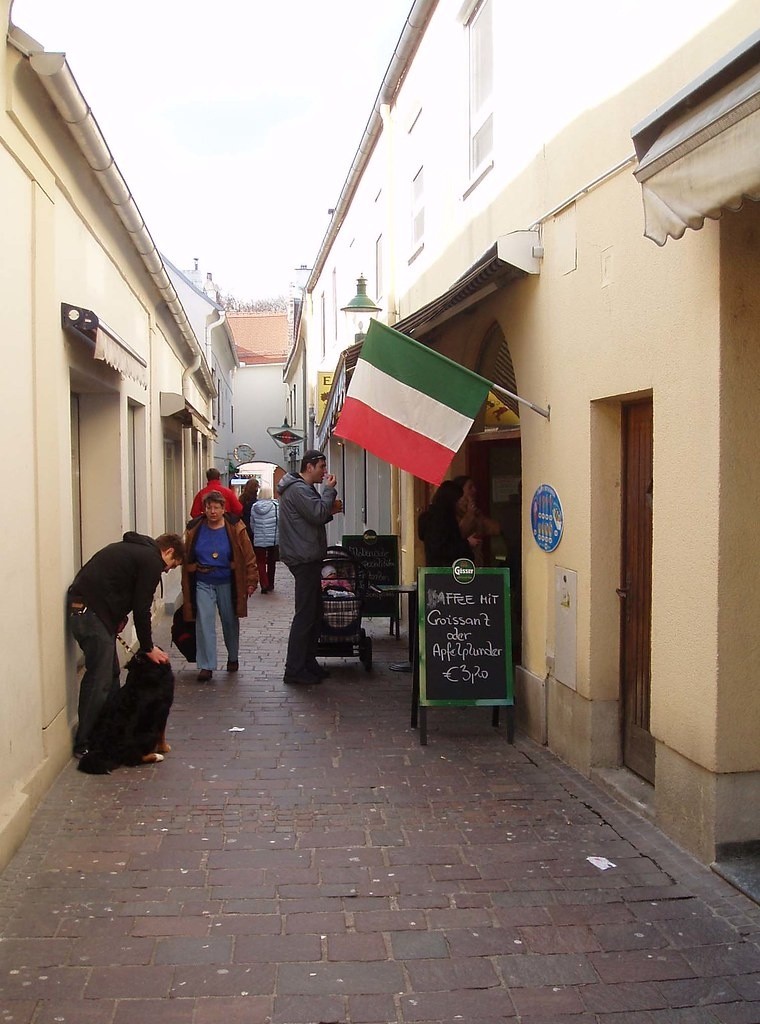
[304,545,373,671]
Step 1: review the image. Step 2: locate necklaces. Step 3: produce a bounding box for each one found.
[212,551,219,558]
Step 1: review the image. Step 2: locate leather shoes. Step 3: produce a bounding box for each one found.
[198,670,213,680]
[227,659,239,672]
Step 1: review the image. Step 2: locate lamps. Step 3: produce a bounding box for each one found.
[340,271,383,312]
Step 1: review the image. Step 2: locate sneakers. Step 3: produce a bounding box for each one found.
[284,660,330,685]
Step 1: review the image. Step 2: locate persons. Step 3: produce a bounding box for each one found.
[66,532,188,759]
[279,449,345,686]
[321,565,351,591]
[181,468,279,682]
[417,475,522,569]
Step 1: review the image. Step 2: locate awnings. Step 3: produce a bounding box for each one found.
[160,392,218,441]
[61,302,148,391]
[632,28,759,246]
[316,227,540,450]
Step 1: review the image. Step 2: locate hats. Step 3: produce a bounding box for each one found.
[321,565,337,578]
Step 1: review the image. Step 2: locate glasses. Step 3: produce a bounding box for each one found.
[311,453,326,461]
[205,505,223,510]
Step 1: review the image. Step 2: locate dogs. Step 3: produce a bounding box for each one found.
[76,644,176,775]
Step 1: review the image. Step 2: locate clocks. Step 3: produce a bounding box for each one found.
[234,444,256,464]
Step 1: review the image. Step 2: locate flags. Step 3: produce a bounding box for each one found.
[331,317,497,490]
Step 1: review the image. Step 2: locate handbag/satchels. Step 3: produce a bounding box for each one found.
[170,605,197,663]
[271,545,280,561]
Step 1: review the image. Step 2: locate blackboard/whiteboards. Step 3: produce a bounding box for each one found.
[341,529,402,617]
[410,567,514,710]
